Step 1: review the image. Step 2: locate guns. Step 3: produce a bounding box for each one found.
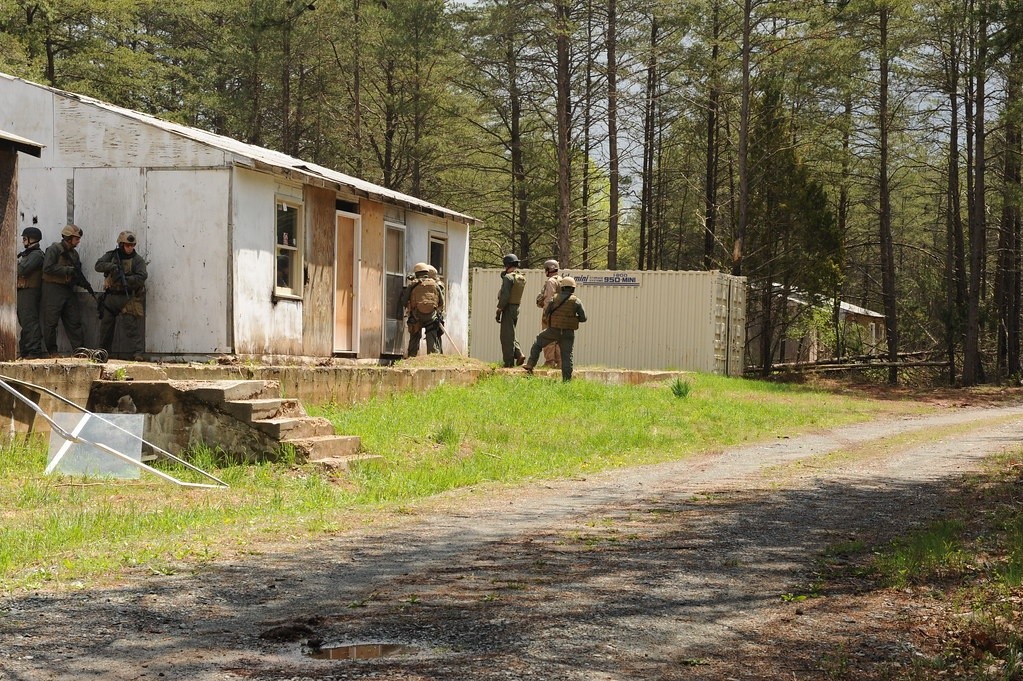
[112,248,130,301]
[61,250,99,305]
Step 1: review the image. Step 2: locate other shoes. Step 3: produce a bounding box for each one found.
[26,354,41,359]
[516,354,525,365]
[521,363,533,370]
[538,361,561,368]
[135,356,143,360]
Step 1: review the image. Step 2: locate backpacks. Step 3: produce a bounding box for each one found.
[411,278,438,314]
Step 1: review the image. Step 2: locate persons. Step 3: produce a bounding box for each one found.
[17,227,45,359]
[42,224,85,358]
[496,254,527,369]
[400,263,445,357]
[94,231,148,361]
[536,260,564,369]
[523,276,587,384]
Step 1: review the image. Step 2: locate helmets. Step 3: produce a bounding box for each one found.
[544,260,559,270]
[414,263,429,272]
[503,254,521,265]
[20,227,42,239]
[116,230,136,247]
[560,277,576,289]
[61,224,83,238]
[428,264,438,279]
